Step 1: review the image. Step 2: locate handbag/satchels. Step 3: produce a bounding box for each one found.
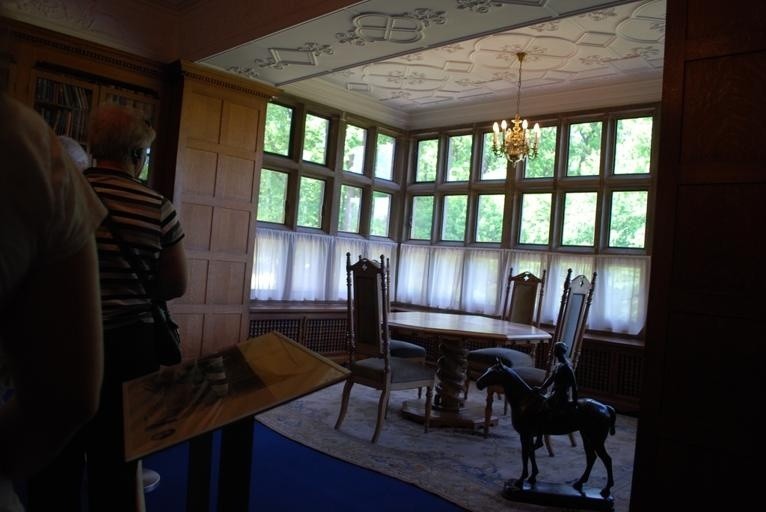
[151,304,182,365]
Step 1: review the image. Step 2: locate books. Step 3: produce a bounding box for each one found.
[34,78,155,168]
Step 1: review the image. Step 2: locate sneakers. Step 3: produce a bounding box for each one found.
[143,467,160,493]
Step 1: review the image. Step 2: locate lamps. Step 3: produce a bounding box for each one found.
[490,51,541,167]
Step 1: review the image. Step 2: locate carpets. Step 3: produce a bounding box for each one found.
[255,365,640,512]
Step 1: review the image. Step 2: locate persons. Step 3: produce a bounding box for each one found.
[531,340,579,450]
[78,99,188,511]
[0,92,112,512]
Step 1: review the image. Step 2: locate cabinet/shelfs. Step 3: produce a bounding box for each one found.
[8,38,166,188]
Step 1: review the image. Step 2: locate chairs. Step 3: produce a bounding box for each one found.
[499,267,548,358]
[481,267,600,455]
[357,255,427,399]
[330,251,432,443]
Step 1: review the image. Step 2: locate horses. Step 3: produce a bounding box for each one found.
[475,356,617,498]
